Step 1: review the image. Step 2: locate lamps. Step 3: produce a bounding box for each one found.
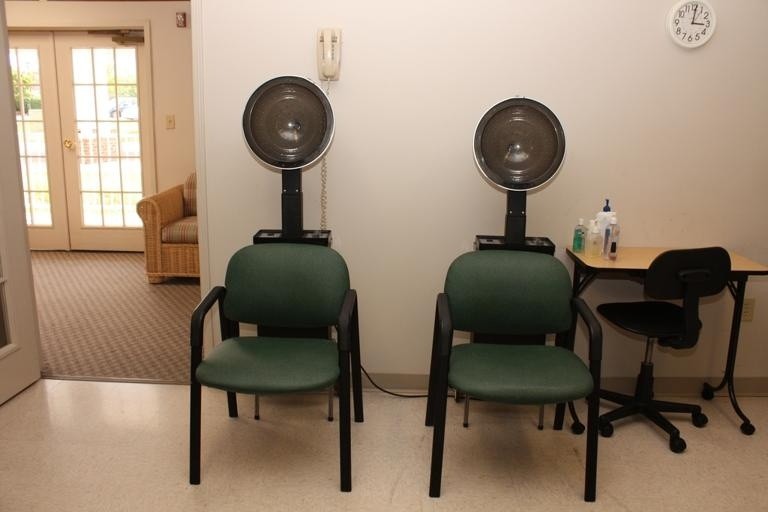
[190,243,363,492]
[426,249,602,502]
[136,172,200,284]
[585,247,731,453]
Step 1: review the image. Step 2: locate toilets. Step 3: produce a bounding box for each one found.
[316,28,341,81]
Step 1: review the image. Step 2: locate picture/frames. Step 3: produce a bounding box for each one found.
[553,246,768,435]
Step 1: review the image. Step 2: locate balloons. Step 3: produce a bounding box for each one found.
[666,0,716,48]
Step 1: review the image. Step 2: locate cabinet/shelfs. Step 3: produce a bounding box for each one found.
[573,217,619,259]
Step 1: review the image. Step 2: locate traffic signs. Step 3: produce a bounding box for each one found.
[741,298,754,322]
